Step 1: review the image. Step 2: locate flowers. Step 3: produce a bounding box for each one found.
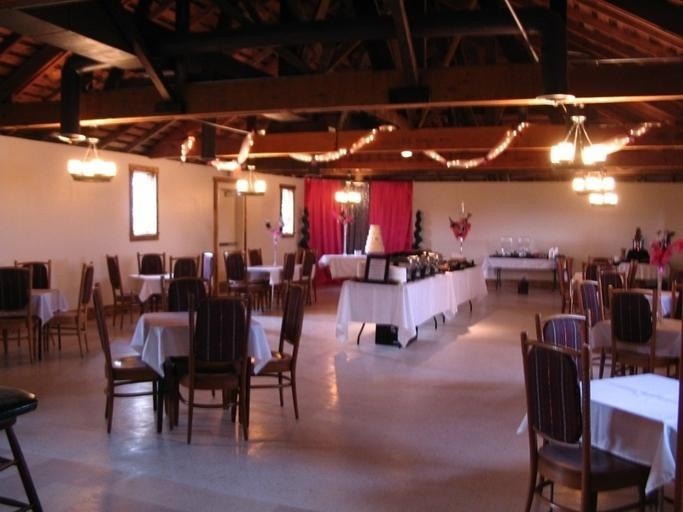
[329,209,356,224]
[265,215,283,246]
[446,213,474,242]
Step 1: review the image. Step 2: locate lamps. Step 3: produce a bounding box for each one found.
[570,163,616,194]
[589,191,618,209]
[550,104,604,169]
[333,168,365,207]
[66,133,118,183]
[235,165,268,197]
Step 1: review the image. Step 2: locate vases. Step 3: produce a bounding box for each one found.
[343,224,347,256]
